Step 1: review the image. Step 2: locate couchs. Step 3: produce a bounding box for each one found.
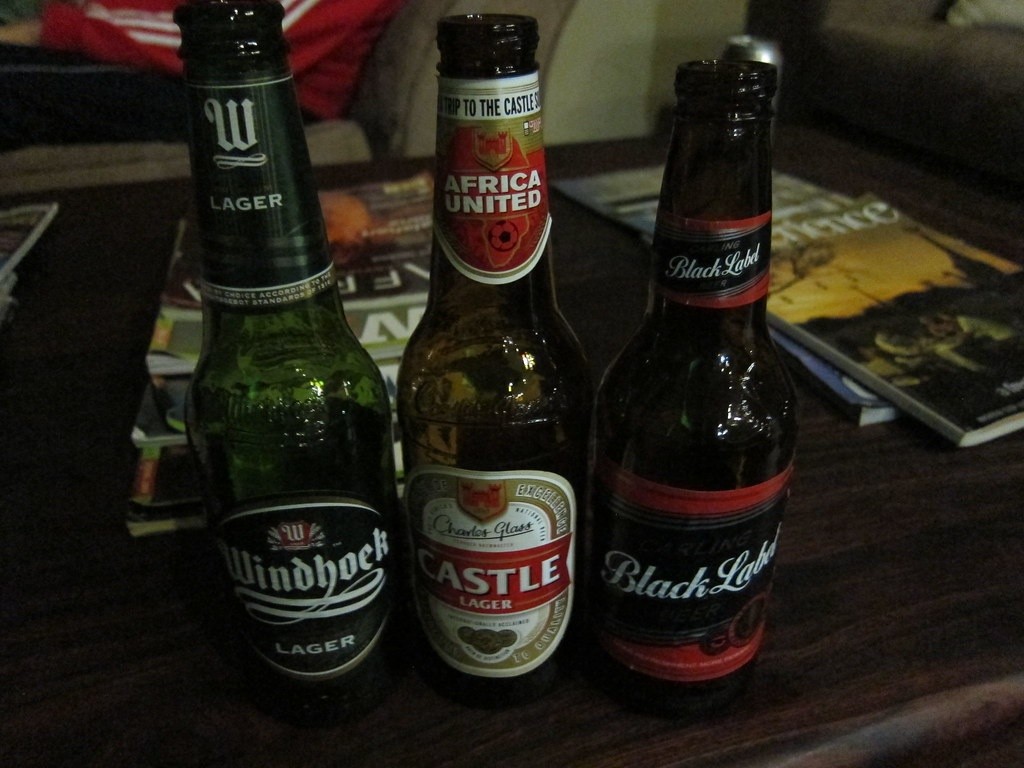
[777,1,1022,197]
[0,2,751,211]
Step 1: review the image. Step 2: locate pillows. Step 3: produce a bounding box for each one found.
[944,2,1023,32]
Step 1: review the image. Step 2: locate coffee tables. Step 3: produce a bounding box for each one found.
[0,129,1024,768]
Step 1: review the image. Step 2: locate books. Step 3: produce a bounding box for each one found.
[2,199,60,335]
[554,155,1024,451]
[125,164,443,531]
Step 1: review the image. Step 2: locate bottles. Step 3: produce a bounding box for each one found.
[168,2,402,722]
[602,53,802,718]
[406,18,596,708]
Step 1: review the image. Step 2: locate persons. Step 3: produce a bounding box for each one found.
[1,2,408,154]
